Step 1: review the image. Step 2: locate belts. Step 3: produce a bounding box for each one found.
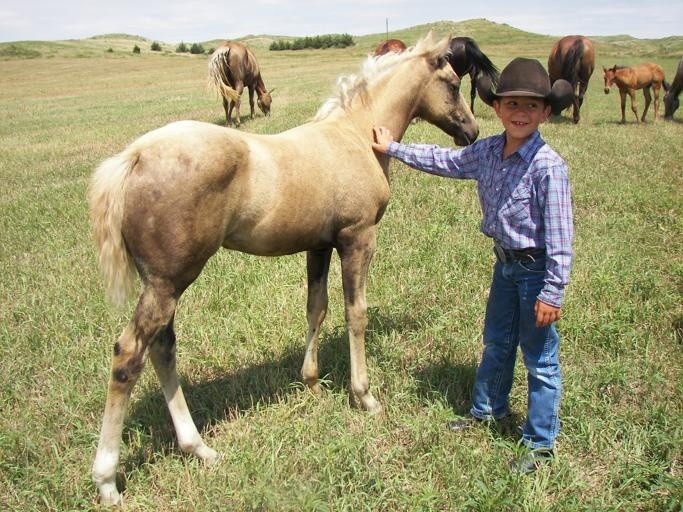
[494,243,545,265]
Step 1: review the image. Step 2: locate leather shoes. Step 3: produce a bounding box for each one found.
[507,447,557,476]
[447,415,480,431]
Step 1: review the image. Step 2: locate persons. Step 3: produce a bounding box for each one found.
[371,57,574,474]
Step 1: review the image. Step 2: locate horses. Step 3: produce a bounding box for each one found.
[664,59,683,120]
[84,29,481,510]
[443,36,502,115]
[547,34,595,124]
[374,39,407,56]
[601,63,671,126]
[206,41,275,128]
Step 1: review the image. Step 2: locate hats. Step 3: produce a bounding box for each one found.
[476,57,575,115]
